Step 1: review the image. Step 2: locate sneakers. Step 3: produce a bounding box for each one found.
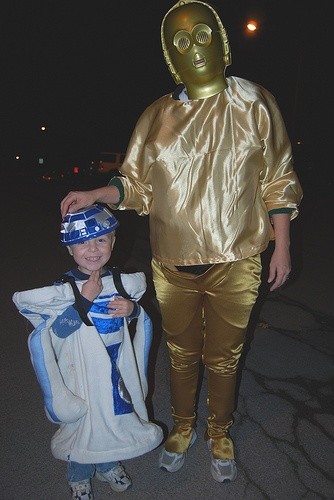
[95,464,132,493]
[67,477,94,500]
[159,429,197,473]
[207,440,237,484]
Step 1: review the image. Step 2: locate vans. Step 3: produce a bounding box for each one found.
[96,152,126,179]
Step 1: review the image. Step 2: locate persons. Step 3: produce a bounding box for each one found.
[12,204,165,500]
[61,0,303,481]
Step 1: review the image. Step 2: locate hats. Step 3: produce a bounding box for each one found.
[58,203,120,246]
